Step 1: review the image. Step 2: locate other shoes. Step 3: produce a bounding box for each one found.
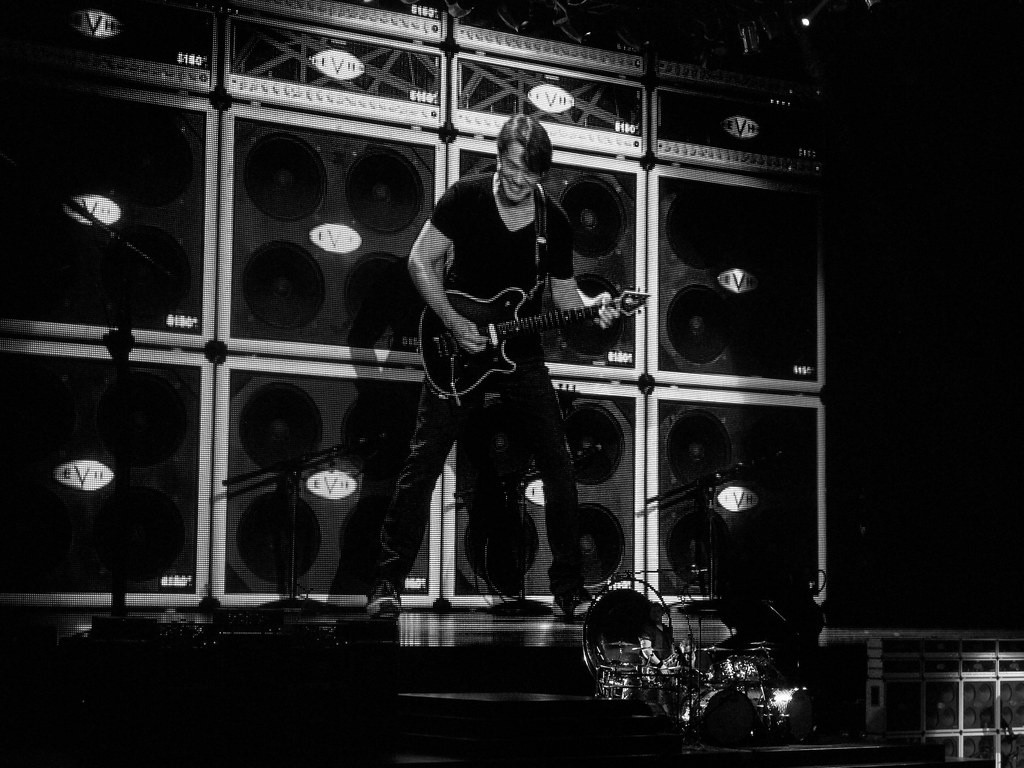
[553,593,596,618]
[365,577,401,618]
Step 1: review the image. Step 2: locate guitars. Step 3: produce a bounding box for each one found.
[417,286,651,397]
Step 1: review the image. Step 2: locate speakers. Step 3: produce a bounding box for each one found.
[0,91,824,626]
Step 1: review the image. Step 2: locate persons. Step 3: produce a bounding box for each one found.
[366,114,621,619]
